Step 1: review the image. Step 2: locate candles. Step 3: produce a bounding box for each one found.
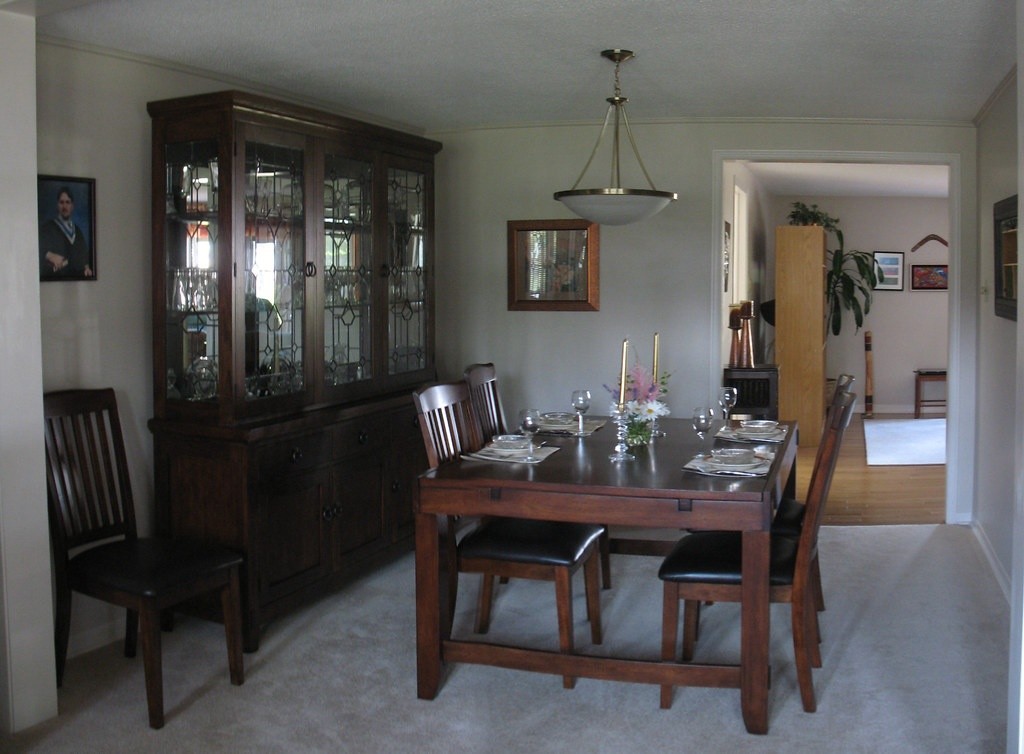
[728,304,742,326]
[652,333,660,385]
[740,300,753,316]
[618,339,629,410]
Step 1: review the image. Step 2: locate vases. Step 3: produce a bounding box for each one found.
[625,414,654,447]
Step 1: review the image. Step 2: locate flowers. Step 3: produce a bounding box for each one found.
[602,345,672,442]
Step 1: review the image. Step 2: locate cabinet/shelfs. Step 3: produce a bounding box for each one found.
[723,366,777,421]
[776,225,827,448]
[145,88,444,632]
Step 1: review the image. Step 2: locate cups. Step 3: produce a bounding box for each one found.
[175,266,218,311]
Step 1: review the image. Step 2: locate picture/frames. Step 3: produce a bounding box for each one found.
[37,172,99,283]
[992,193,1017,322]
[506,218,601,311]
[872,251,904,291]
[909,263,948,292]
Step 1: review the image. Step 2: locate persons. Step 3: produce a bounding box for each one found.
[39,185,92,281]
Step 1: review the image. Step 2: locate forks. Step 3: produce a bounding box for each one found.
[696,467,758,476]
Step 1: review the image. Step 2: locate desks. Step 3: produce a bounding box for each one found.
[914,369,947,418]
[414,414,802,737]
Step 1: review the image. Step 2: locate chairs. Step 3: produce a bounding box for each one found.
[411,376,602,690]
[657,390,856,714]
[464,359,614,622]
[41,387,249,729]
[689,374,855,645]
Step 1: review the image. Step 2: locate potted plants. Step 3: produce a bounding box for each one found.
[786,201,884,338]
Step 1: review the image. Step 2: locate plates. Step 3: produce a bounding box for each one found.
[486,441,535,455]
[187,357,217,398]
[736,429,784,438]
[706,458,762,470]
[538,421,578,430]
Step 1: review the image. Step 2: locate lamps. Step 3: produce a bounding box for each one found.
[553,49,677,227]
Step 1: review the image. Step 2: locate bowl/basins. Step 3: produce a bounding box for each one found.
[742,420,778,433]
[712,449,754,464]
[493,435,529,448]
[542,412,574,424]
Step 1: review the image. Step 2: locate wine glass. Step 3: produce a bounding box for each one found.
[246,176,272,216]
[572,390,591,436]
[692,408,714,458]
[717,387,737,431]
[519,409,543,463]
[324,266,359,304]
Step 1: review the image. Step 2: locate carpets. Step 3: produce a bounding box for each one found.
[863,417,948,465]
